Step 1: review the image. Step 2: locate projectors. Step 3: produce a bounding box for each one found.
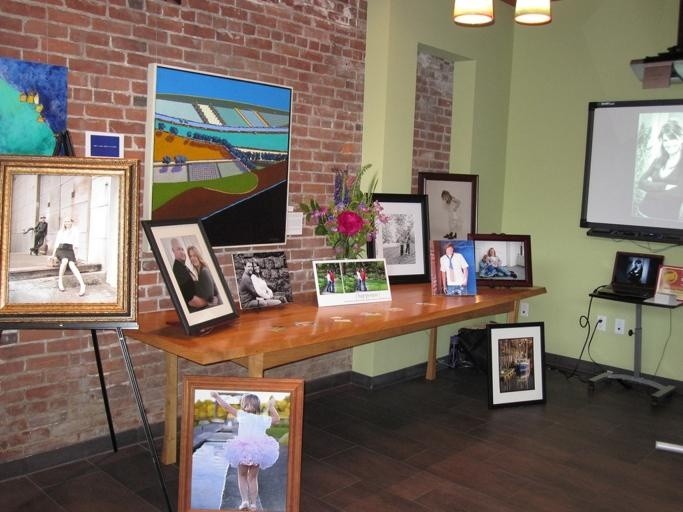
[629,50,682,91]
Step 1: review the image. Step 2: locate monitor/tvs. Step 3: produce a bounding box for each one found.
[579,98,683,244]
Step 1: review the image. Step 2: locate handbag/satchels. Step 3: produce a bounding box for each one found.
[456,326,488,372]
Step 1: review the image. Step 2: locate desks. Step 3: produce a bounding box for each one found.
[588,290,683,407]
[121,281,548,464]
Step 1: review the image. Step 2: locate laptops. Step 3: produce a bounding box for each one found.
[596,251,664,302]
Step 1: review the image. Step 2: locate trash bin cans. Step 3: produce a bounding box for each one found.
[454,328,492,393]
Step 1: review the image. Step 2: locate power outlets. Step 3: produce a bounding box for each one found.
[595,315,607,331]
[614,318,625,335]
[520,303,528,316]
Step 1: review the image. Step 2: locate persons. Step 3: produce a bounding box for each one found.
[400,232,411,255]
[239,260,286,307]
[479,248,517,278]
[627,260,643,283]
[29,216,48,256]
[210,391,281,510]
[327,269,335,293]
[170,238,222,313]
[50,216,86,296]
[440,243,469,295]
[441,191,460,239]
[637,119,683,220]
[357,267,367,291]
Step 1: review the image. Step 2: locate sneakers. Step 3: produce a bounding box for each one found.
[239,501,257,512]
[509,270,517,278]
[58,279,85,296]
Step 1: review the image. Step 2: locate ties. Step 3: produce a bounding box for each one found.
[446,254,454,281]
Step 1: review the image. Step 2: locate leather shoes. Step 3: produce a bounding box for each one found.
[30,249,38,255]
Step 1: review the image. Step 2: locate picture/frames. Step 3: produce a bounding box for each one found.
[418,172,479,240]
[485,321,547,408]
[312,258,392,307]
[143,63,293,253]
[175,376,305,512]
[365,194,431,286]
[231,251,293,310]
[467,232,535,286]
[1,154,139,323]
[139,219,240,336]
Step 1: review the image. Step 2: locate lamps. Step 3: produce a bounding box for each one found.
[453,0,552,26]
